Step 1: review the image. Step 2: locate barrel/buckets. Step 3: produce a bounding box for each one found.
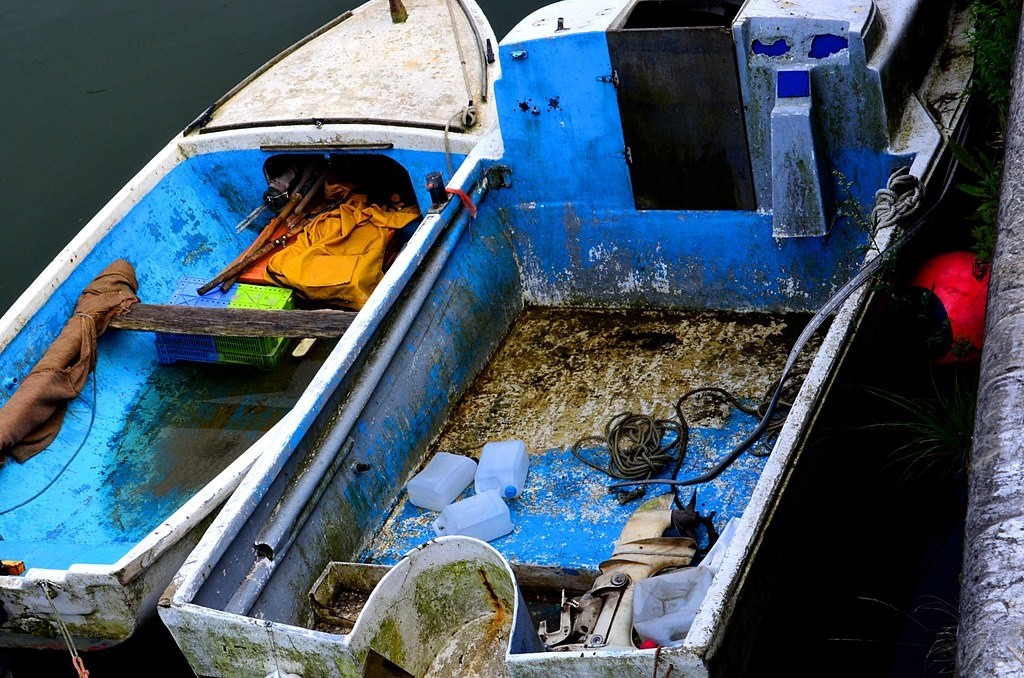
[632,566,711,648]
[407,452,478,512]
[474,440,529,500]
[432,490,513,546]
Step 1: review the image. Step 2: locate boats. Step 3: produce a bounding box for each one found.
[0,0,502,654]
[156,0,978,678]
